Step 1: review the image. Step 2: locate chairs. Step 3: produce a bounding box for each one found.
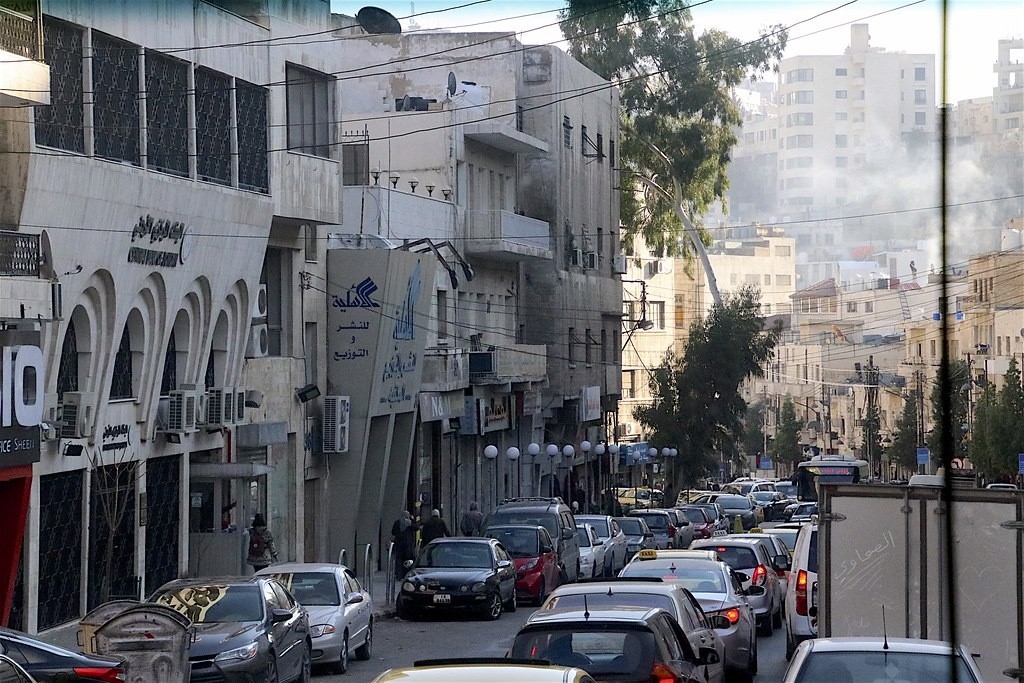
[736,553,757,567]
[538,519,554,535]
[653,517,663,527]
[610,631,657,667]
[527,533,537,552]
[540,633,591,664]
[579,533,587,546]
[497,533,515,552]
[697,580,718,592]
[808,658,856,683]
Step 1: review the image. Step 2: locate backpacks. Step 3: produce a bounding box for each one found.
[248,527,269,556]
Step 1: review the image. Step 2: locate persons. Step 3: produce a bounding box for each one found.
[247,513,279,573]
[725,469,744,483]
[574,484,585,514]
[713,481,720,490]
[745,473,749,477]
[460,502,485,537]
[391,510,420,581]
[419,509,453,553]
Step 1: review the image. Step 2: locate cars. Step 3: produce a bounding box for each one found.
[394,498,695,621]
[511,526,799,683]
[370,651,599,683]
[602,487,666,510]
[0,626,130,683]
[678,477,817,531]
[782,475,1024,683]
[146,561,374,683]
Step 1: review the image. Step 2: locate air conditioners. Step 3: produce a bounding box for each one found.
[253,284,267,319]
[570,249,583,267]
[168,389,197,431]
[243,324,269,358]
[653,260,663,274]
[59,391,95,439]
[208,386,234,426]
[322,395,350,453]
[613,256,627,273]
[232,386,246,424]
[587,253,599,270]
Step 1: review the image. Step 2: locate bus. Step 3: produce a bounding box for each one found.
[790,453,871,502]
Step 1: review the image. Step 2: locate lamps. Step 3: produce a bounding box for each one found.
[441,185,452,200]
[425,181,436,197]
[389,172,400,188]
[370,167,382,185]
[621,320,654,335]
[245,389,265,408]
[408,177,419,193]
[295,383,321,404]
[62,440,84,457]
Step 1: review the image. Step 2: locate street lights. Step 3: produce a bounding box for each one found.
[608,444,618,517]
[484,445,498,512]
[546,444,558,498]
[595,444,605,515]
[579,440,591,515]
[648,447,658,509]
[528,443,540,497]
[790,396,826,456]
[563,445,574,510]
[632,450,640,509]
[506,446,519,498]
[961,379,990,481]
[900,387,928,475]
[661,448,678,507]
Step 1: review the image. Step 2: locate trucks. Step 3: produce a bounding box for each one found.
[731,454,777,478]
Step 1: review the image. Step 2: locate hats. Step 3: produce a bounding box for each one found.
[251,513,267,526]
